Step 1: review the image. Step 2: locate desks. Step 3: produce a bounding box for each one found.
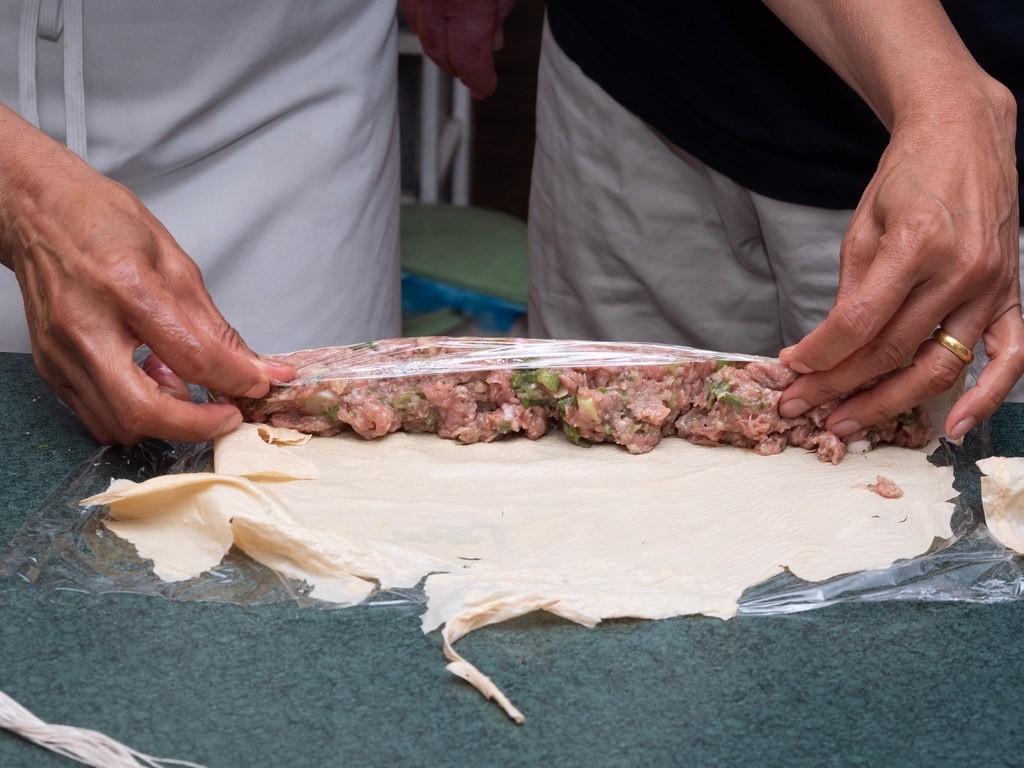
[0,348,1024,768]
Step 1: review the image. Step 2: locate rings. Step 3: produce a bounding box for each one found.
[932,328,975,364]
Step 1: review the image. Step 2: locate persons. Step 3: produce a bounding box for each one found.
[1,0,1024,449]
[394,0,1024,401]
